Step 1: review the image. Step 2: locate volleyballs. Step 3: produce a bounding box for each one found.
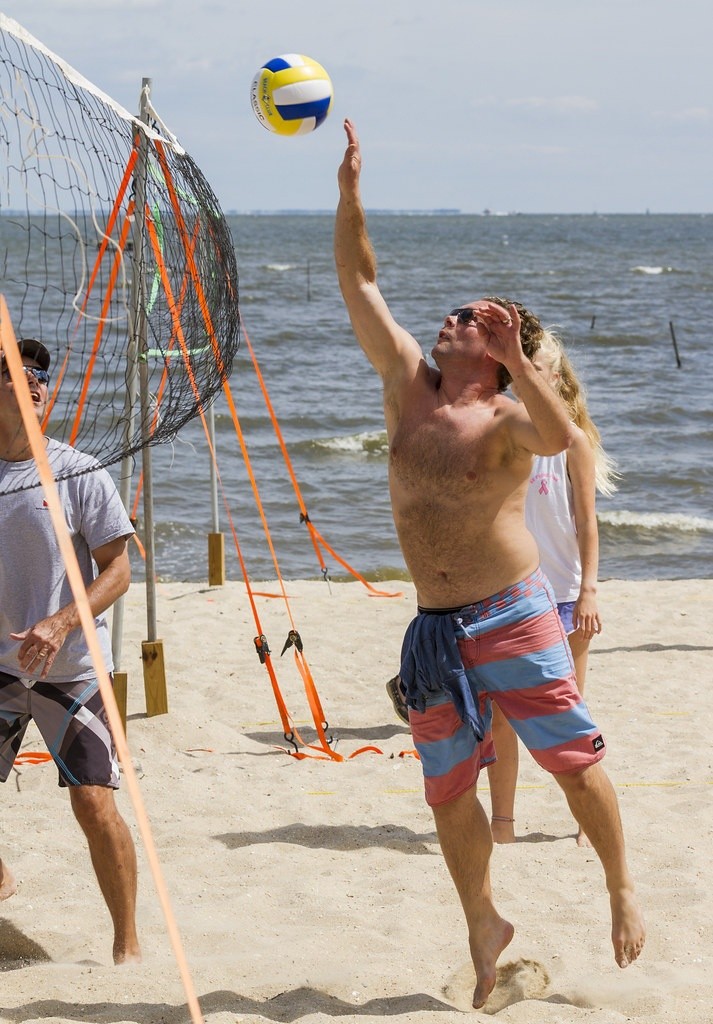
[248,52,336,138]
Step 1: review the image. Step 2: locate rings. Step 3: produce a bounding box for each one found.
[39,652,45,657]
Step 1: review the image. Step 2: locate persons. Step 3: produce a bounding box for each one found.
[0,339,146,967]
[337,118,646,1010]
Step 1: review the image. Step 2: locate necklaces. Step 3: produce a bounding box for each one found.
[502,316,511,323]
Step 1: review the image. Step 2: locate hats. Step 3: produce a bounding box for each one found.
[0,339,50,370]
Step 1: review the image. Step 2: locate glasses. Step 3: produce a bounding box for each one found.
[452,309,476,327]
[2,367,53,386]
